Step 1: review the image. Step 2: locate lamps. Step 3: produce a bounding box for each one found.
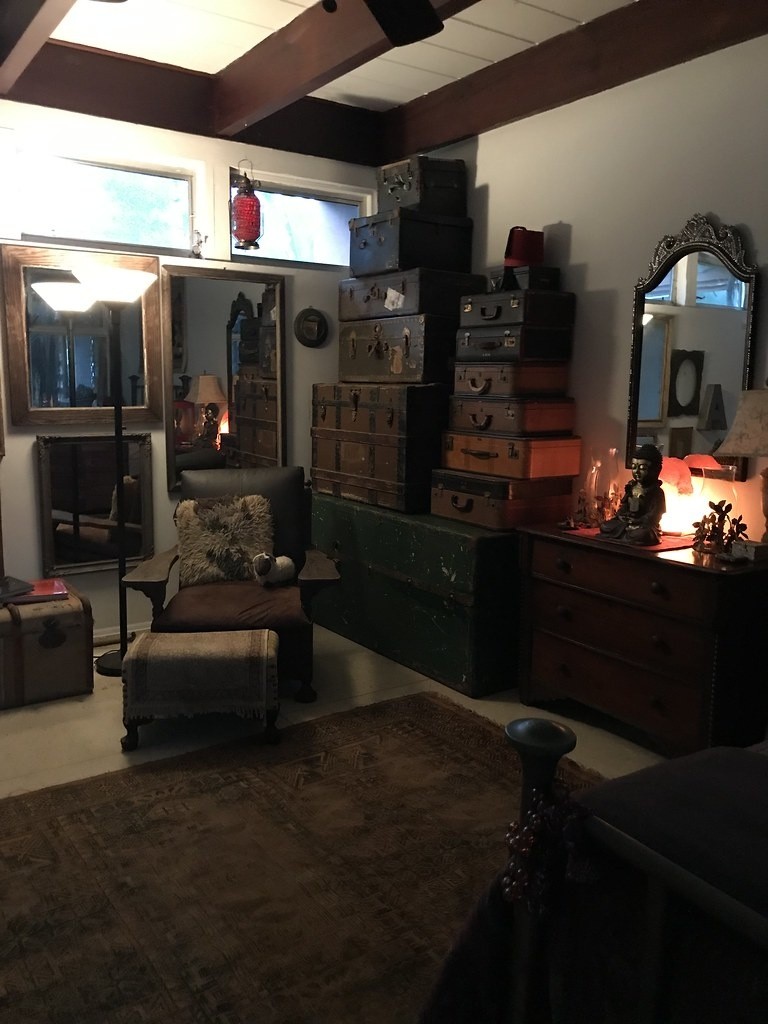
[712,387,768,545]
[183,369,227,436]
[659,458,694,534]
[684,454,720,490]
[27,281,99,407]
[71,263,162,675]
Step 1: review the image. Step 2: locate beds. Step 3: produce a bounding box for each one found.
[416,718,768,1024]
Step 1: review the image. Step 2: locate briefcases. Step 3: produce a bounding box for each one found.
[429,266,584,530]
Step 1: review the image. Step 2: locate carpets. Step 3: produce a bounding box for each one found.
[0,690,609,1024]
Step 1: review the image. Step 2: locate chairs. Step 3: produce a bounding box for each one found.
[51,476,140,566]
[122,465,342,703]
[175,449,223,477]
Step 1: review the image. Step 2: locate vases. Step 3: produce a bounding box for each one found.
[697,465,739,540]
[584,447,622,526]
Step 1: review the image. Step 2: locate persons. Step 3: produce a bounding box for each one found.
[192,404,218,447]
[601,444,666,545]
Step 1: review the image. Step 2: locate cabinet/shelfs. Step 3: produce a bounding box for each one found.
[517,524,768,755]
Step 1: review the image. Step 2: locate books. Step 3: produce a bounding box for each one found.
[0,575,69,602]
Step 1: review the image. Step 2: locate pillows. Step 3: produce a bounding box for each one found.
[109,473,139,550]
[173,495,276,587]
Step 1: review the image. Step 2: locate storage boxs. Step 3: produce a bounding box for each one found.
[0,578,96,710]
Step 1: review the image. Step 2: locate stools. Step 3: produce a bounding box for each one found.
[121,628,282,752]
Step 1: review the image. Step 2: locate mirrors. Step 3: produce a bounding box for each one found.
[36,434,155,580]
[160,265,287,492]
[624,212,758,483]
[1,243,166,428]
[226,291,254,427]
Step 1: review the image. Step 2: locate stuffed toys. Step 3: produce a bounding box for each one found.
[252,552,295,587]
